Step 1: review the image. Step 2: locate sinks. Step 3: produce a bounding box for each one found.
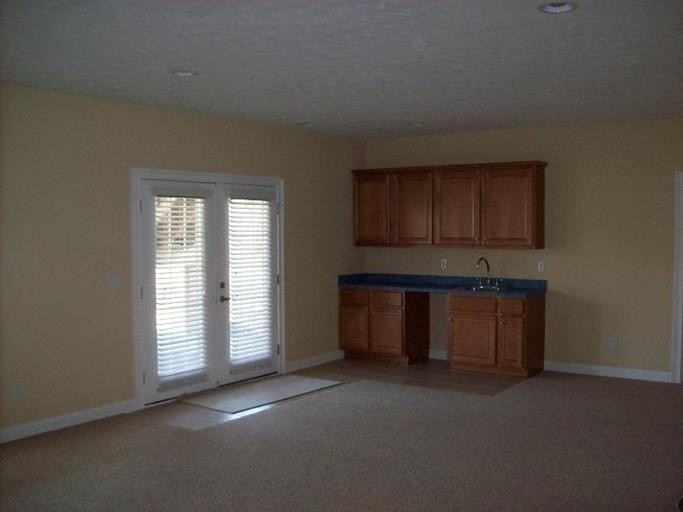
[466,285,508,292]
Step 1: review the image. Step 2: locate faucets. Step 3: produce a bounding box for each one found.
[476,256,491,281]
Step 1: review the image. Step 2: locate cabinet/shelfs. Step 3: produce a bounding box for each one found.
[447,293,546,379]
[339,289,430,367]
[352,161,551,250]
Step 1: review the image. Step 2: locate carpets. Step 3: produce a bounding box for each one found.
[177,371,343,415]
